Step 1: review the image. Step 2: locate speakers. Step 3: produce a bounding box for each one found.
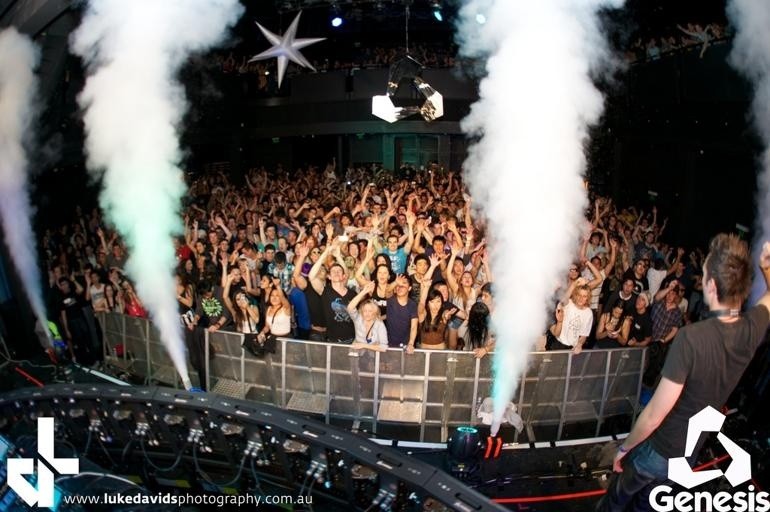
[345,76,354,92]
[72,317,103,367]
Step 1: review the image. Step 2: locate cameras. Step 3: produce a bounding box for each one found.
[411,182,417,185]
[368,182,375,186]
[347,181,351,185]
[339,236,348,241]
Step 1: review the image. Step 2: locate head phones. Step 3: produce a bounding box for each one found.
[699,309,741,320]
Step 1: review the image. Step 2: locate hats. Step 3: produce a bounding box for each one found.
[639,291,651,307]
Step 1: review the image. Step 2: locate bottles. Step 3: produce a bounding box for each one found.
[111,342,135,362]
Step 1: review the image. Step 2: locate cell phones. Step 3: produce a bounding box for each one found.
[611,332,618,334]
[240,294,245,300]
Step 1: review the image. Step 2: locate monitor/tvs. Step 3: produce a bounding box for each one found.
[255,79,291,97]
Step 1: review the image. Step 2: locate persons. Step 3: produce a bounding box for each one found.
[34,155,708,416]
[190,19,732,88]
[593,229,770,512]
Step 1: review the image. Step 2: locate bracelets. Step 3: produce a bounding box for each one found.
[619,445,631,454]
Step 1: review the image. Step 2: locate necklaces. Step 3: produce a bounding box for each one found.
[708,306,740,320]
